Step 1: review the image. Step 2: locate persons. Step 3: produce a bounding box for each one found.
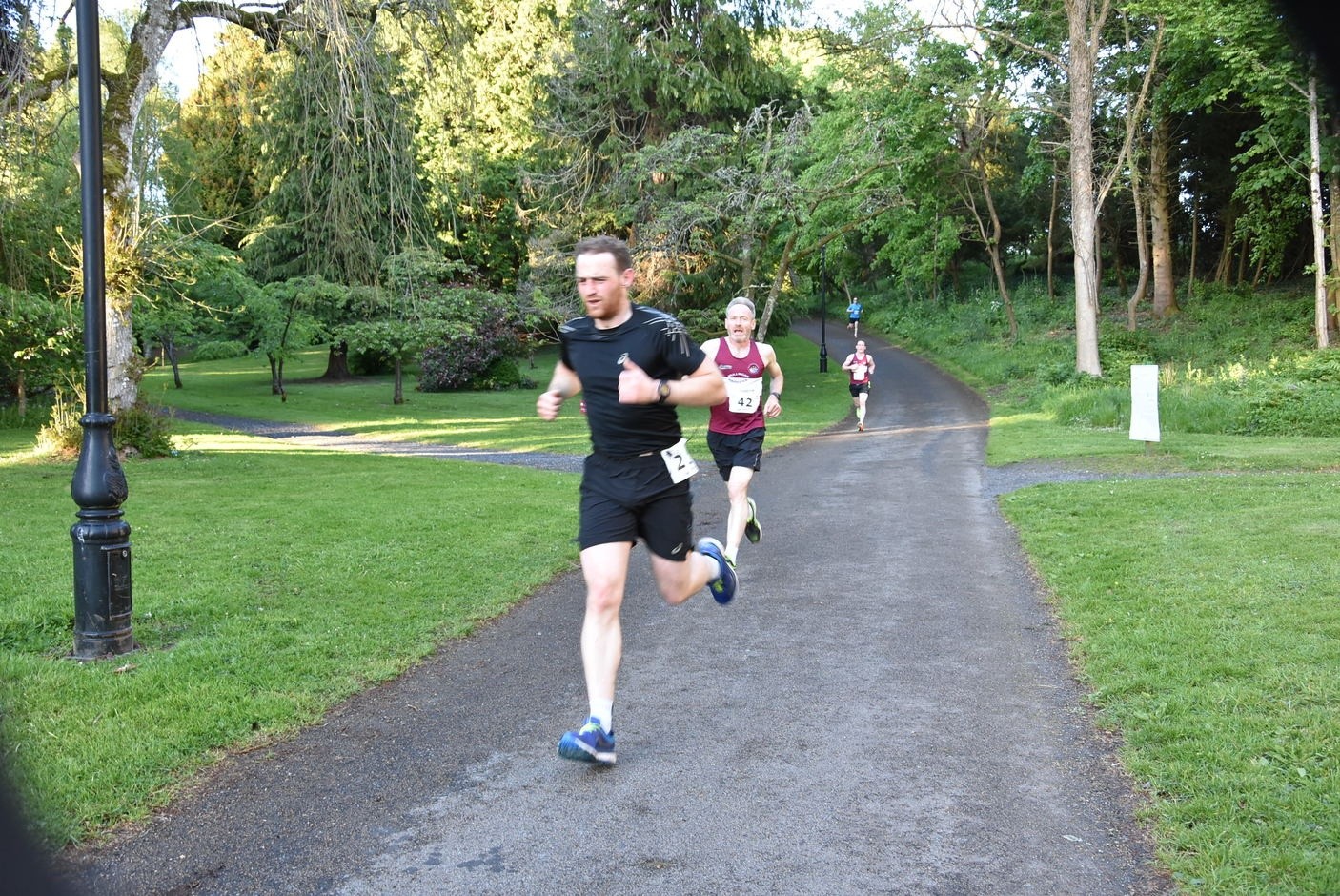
[535,234,738,765]
[846,297,864,337]
[842,340,876,431]
[691,296,783,565]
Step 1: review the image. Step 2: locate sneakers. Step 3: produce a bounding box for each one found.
[557,715,617,766]
[744,496,762,544]
[723,553,737,572]
[697,537,739,607]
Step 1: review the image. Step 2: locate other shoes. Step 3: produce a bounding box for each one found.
[857,422,863,431]
[856,407,861,419]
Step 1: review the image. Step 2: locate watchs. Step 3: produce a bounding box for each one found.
[658,379,670,406]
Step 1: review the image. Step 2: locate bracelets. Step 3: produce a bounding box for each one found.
[770,392,781,400]
